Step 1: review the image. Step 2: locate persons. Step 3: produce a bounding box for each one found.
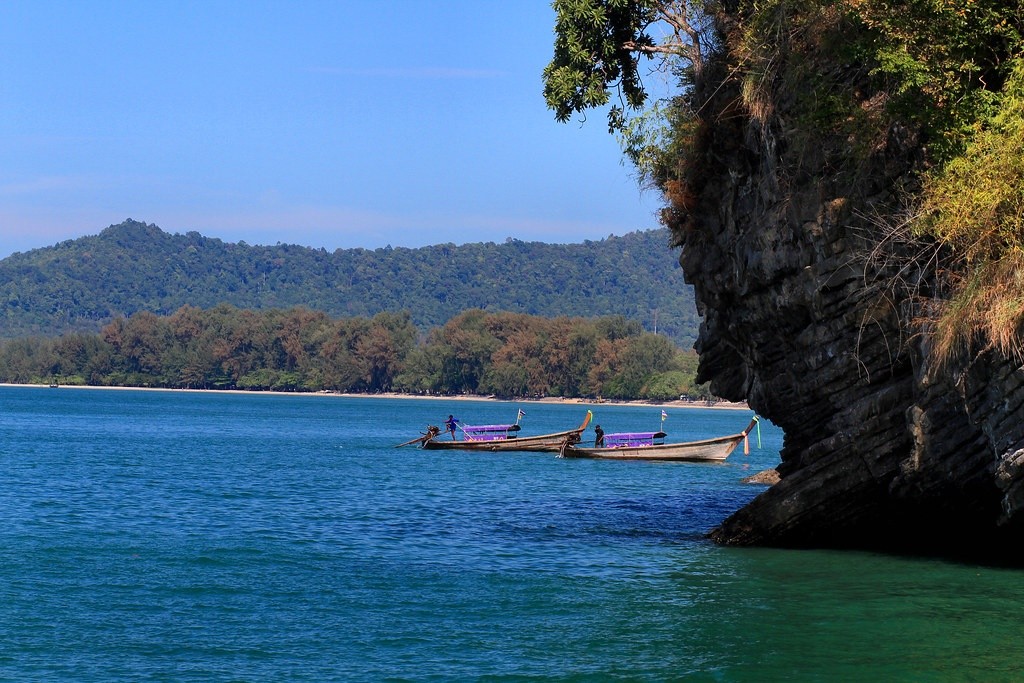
[443,415,459,441]
[595,425,604,448]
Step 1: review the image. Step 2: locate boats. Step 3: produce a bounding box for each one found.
[421,409,592,453]
[564,415,758,461]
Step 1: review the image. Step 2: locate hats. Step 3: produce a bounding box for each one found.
[596,425,600,427]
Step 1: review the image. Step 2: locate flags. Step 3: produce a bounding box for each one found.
[517,409,525,420]
[662,410,667,421]
